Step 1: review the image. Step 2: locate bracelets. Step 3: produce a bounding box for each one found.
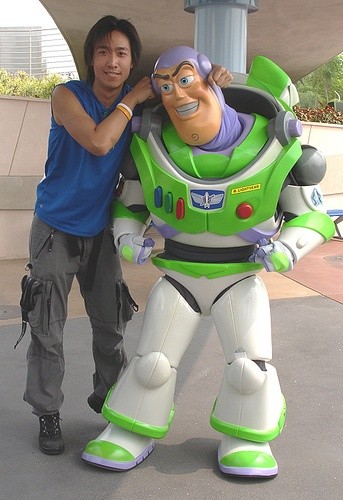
[117,102,133,117]
[117,105,131,120]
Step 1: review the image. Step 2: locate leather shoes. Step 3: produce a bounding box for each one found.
[38,411,65,455]
[86,392,106,413]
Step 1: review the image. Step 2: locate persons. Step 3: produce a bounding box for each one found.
[14,15,234,456]
[82,44,336,479]
